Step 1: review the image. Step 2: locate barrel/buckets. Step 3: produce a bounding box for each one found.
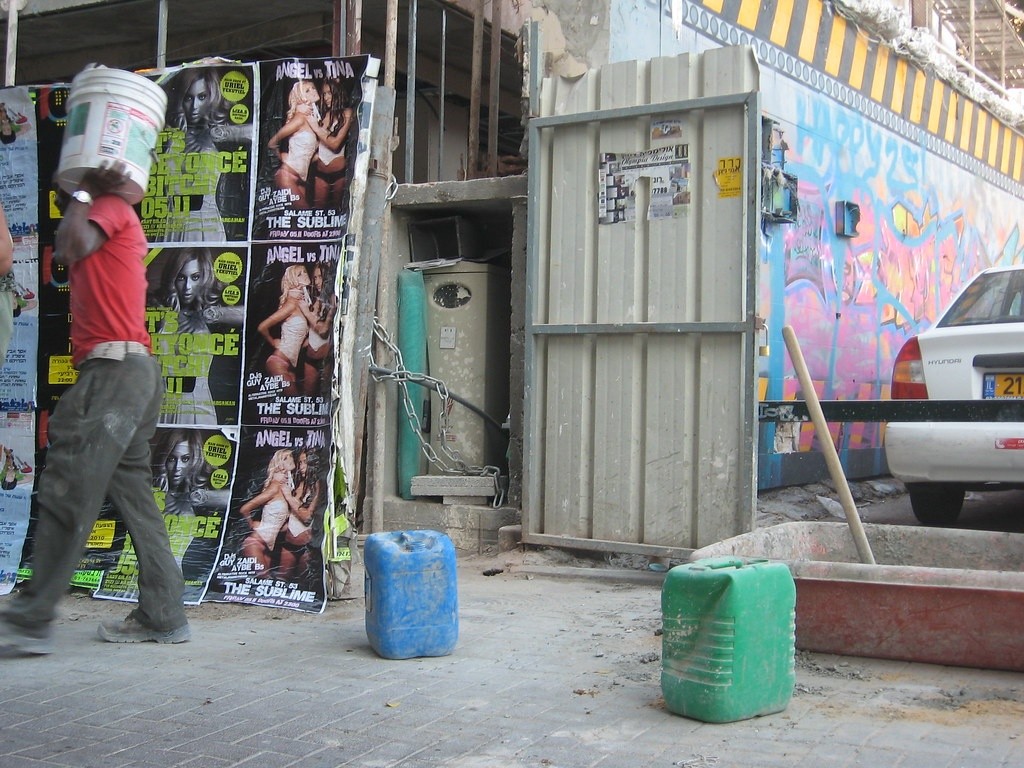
[57,67,167,202]
[363,529,457,660]
[660,556,796,723]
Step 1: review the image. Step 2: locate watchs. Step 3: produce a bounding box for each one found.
[72,191,95,209]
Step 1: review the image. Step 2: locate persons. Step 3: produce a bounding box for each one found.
[139,430,233,581]
[153,69,253,246]
[150,247,245,429]
[235,72,356,596]
[0,102,18,144]
[1,157,191,653]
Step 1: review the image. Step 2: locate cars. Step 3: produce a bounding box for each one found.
[884,264,1023,528]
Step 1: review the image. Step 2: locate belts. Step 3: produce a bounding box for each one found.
[74,341,150,367]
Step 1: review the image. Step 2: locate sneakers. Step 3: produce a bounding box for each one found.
[97,613,191,645]
[0,608,54,654]
